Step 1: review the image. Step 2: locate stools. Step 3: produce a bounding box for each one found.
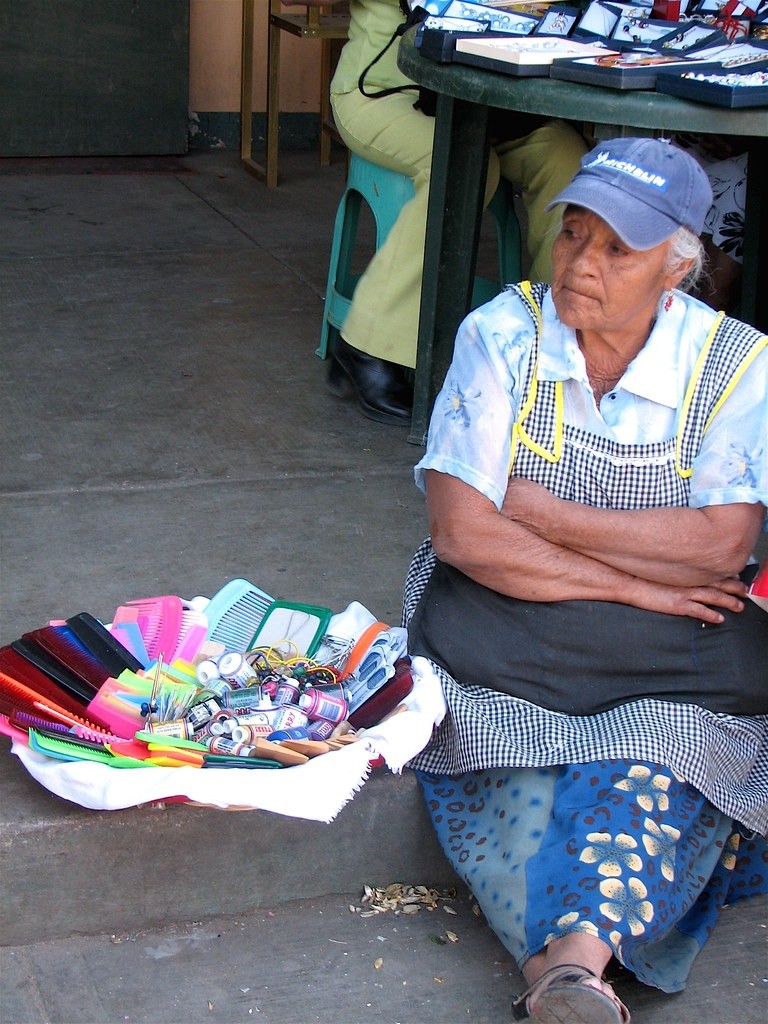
[313,149,521,359]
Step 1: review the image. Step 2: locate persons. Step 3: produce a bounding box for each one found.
[329,1,588,423]
[588,0,768,311]
[401,137,768,1024]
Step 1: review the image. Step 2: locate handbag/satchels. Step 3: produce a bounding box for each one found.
[359,5,553,147]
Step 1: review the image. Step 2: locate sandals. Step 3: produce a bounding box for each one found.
[511,963,631,1024]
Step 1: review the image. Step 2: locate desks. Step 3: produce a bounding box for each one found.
[394,15,768,448]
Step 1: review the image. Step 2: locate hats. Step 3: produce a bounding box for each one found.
[545,138,712,252]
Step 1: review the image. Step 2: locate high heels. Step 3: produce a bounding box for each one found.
[325,330,415,427]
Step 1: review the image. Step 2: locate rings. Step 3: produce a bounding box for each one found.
[680,13,717,23]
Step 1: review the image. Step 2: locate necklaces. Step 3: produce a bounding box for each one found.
[595,54,705,68]
[663,28,705,49]
[696,41,768,66]
